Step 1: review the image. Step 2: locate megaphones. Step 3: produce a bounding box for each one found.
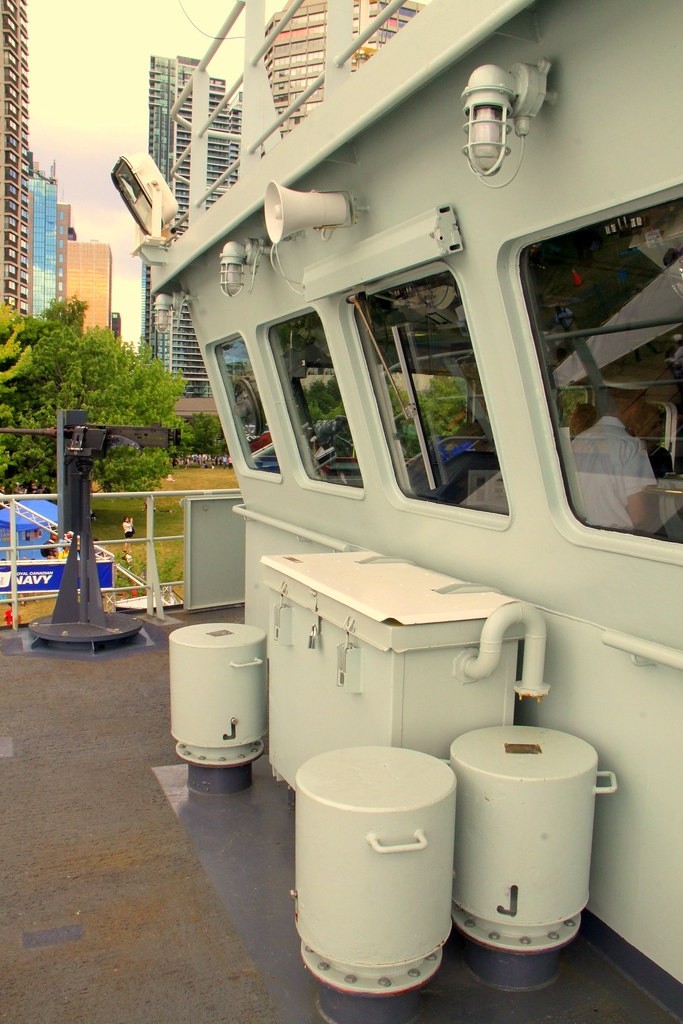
[263,180,350,243]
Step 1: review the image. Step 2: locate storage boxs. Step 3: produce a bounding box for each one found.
[257,548,523,794]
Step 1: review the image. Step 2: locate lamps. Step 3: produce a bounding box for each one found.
[219,240,275,297]
[153,292,190,332]
[460,58,557,187]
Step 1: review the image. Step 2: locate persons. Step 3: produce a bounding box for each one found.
[122,515,135,553]
[180,453,232,470]
[40,530,80,562]
[0,479,51,494]
[568,383,673,538]
